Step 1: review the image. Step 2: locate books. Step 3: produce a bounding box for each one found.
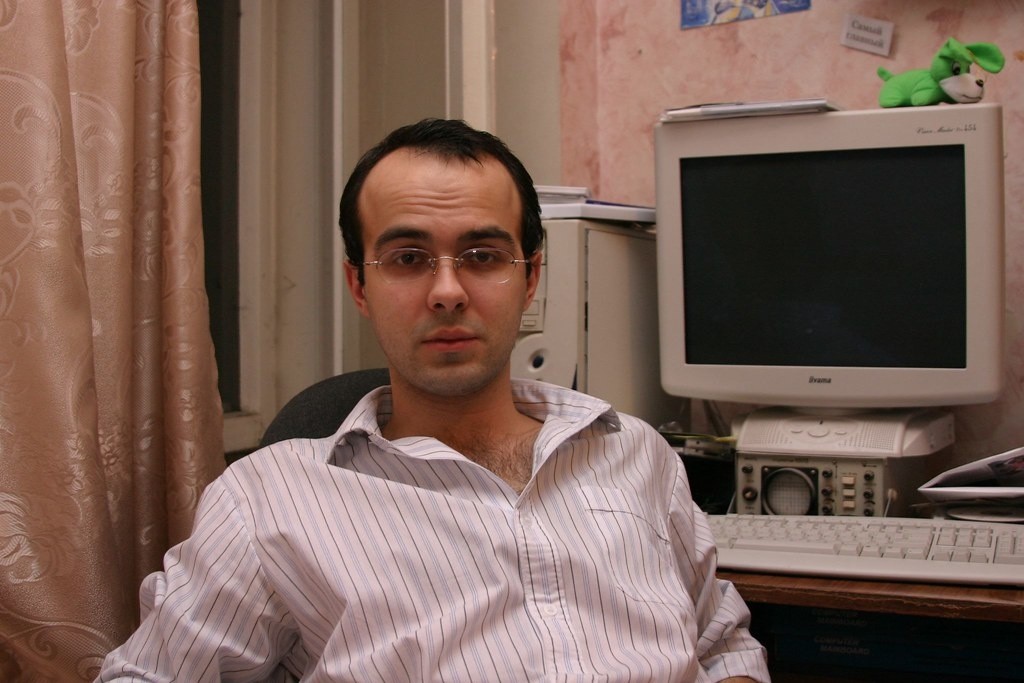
[659,98,835,126]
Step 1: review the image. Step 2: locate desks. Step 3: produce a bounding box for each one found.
[717,569,1024,621]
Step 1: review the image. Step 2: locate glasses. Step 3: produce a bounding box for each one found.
[361,247,543,284]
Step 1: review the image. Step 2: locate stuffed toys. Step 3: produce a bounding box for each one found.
[877,38,1004,108]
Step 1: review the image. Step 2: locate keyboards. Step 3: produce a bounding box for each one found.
[703,512,1023,584]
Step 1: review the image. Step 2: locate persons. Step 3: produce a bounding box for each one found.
[94,117,769,683]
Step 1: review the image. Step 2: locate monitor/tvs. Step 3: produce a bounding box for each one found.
[649,102,1004,458]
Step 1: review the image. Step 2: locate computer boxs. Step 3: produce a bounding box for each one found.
[509,219,693,443]
